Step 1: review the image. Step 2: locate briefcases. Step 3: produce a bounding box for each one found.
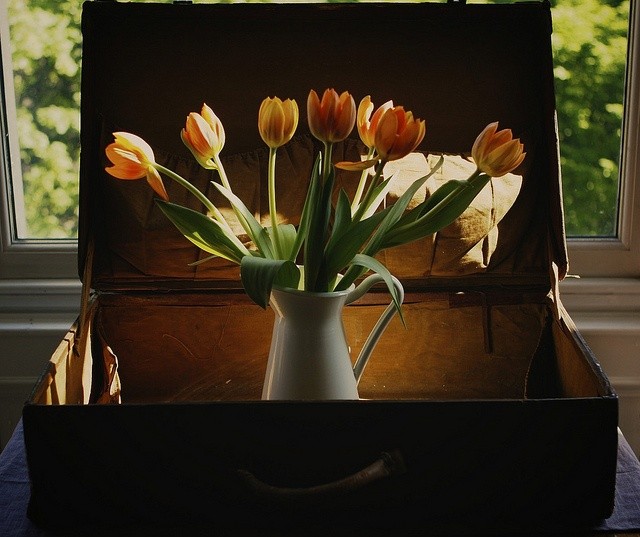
[24,2,620,532]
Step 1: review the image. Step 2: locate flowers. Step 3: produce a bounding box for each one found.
[104,86,528,329]
